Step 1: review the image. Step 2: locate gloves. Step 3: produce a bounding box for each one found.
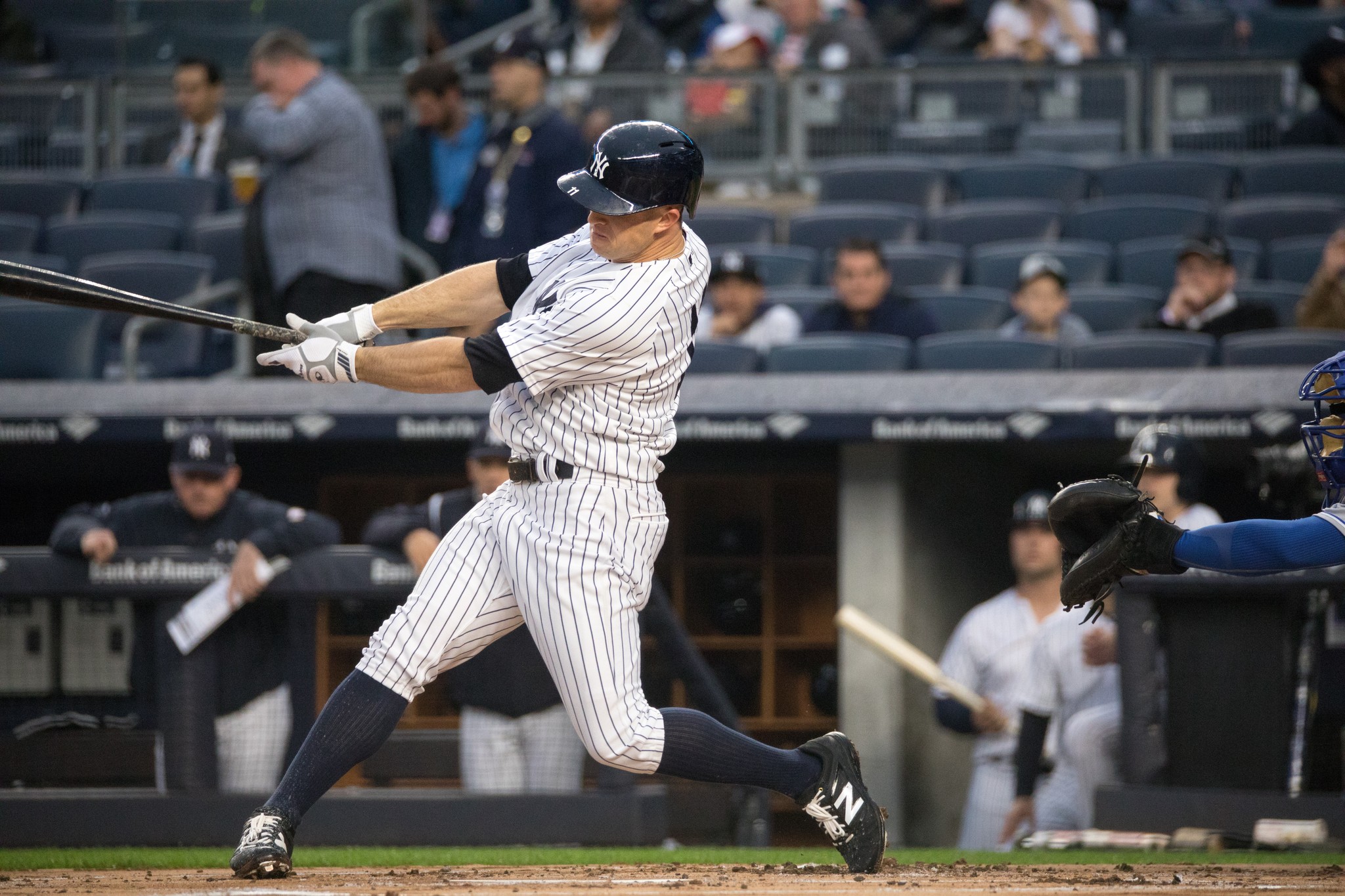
[255,313,359,384]
[282,302,383,350]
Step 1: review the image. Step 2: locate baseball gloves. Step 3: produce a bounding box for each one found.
[1046,466,1188,612]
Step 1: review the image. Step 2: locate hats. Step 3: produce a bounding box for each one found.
[1019,250,1069,288]
[710,251,765,289]
[167,429,235,479]
[469,424,514,459]
[1177,233,1235,268]
[1010,490,1052,523]
[475,32,546,74]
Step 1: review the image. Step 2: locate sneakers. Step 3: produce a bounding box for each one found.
[795,731,888,872]
[229,802,298,879]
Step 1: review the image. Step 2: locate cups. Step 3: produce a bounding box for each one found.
[230,157,262,204]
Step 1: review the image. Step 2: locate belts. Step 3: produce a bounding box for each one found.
[508,457,573,484]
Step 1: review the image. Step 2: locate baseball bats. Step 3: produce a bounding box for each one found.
[0,260,378,351]
[831,601,1017,741]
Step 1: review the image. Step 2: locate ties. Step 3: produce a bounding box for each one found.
[186,133,205,177]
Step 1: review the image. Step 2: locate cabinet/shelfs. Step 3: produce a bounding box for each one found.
[308,476,832,846]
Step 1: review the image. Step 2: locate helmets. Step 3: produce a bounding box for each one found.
[556,121,703,220]
[1122,424,1192,474]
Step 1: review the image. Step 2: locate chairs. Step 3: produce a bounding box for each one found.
[1,0,1345,378]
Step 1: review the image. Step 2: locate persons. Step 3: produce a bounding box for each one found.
[228,120,887,876]
[47,1,1345,879]
